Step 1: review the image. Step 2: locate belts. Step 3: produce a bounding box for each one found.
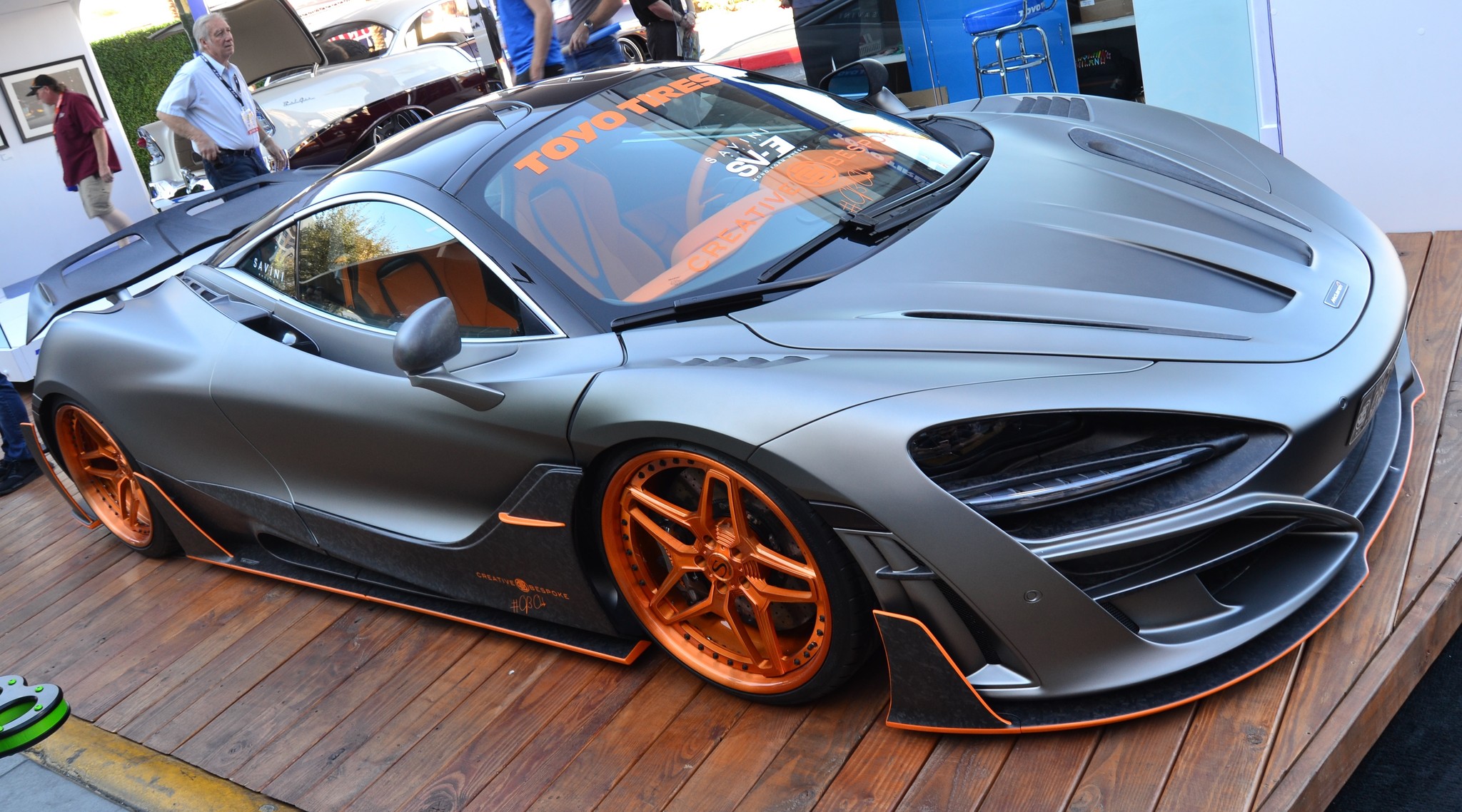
[227,148,257,157]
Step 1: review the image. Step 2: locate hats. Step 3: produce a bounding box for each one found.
[24,75,53,96]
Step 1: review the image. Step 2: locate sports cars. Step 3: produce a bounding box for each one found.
[23,58,1421,733]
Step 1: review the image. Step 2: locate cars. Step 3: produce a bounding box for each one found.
[135,0,506,214]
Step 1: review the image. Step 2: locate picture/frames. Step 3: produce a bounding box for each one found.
[0,124,10,151]
[0,54,111,143]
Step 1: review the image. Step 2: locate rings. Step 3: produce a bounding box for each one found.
[692,23,695,25]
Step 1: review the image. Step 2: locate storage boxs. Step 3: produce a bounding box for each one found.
[1078,0,1134,24]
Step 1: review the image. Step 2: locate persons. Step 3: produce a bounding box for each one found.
[779,0,864,93]
[25,74,140,251]
[155,11,292,207]
[494,0,568,92]
[627,0,703,66]
[0,366,57,501]
[566,0,629,72]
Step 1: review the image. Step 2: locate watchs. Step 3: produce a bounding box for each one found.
[688,11,697,19]
[583,18,594,32]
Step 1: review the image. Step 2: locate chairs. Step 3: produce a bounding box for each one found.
[337,201,522,332]
[961,0,1059,99]
[499,132,671,300]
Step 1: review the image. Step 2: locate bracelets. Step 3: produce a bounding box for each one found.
[678,14,684,24]
[260,134,273,145]
